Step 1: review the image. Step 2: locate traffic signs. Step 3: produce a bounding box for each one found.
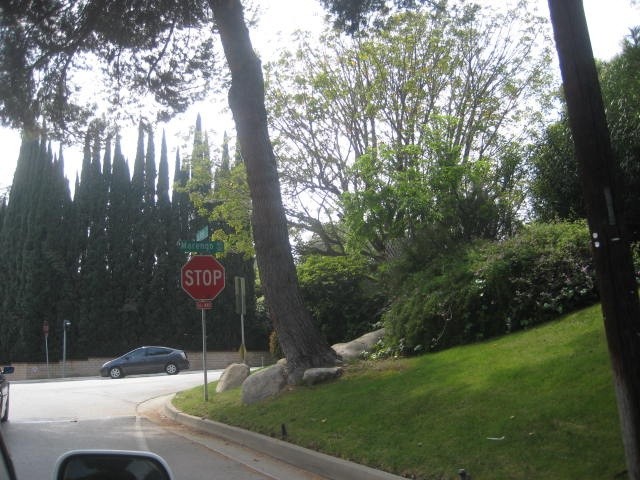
[178,241,224,252]
[196,225,208,241]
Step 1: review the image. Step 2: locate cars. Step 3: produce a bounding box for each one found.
[0,367,14,421]
[100,346,189,378]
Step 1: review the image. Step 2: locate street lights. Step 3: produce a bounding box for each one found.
[63,320,71,377]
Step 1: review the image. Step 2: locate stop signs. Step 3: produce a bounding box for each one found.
[181,255,225,301]
